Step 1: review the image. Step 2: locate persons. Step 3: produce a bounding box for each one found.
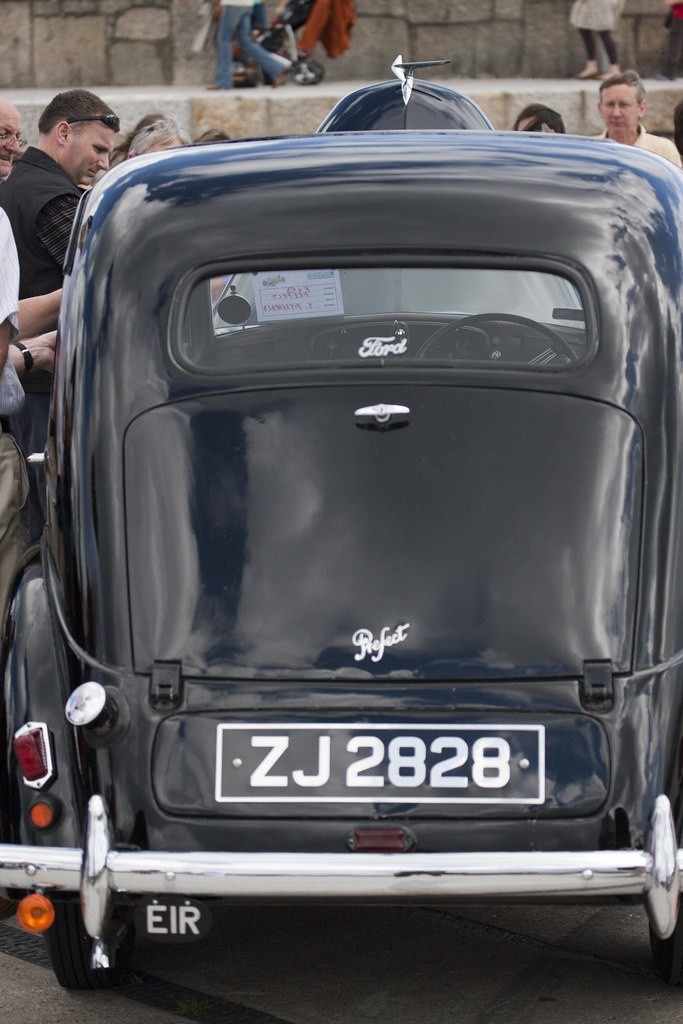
[569,0,625,80]
[655,0,683,79]
[513,104,567,134]
[597,70,682,168]
[207,0,294,90]
[1,91,232,648]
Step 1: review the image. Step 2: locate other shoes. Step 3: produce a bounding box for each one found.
[204,81,222,89]
[273,67,294,91]
[573,67,598,79]
[654,73,670,82]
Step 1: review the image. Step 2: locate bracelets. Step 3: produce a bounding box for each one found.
[13,343,34,373]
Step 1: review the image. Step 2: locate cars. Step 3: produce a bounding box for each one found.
[1,75,683,989]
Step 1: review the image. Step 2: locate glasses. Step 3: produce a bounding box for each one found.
[67,115,121,132]
[0,135,27,149]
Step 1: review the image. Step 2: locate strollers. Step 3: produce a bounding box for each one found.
[227,0,331,85]
